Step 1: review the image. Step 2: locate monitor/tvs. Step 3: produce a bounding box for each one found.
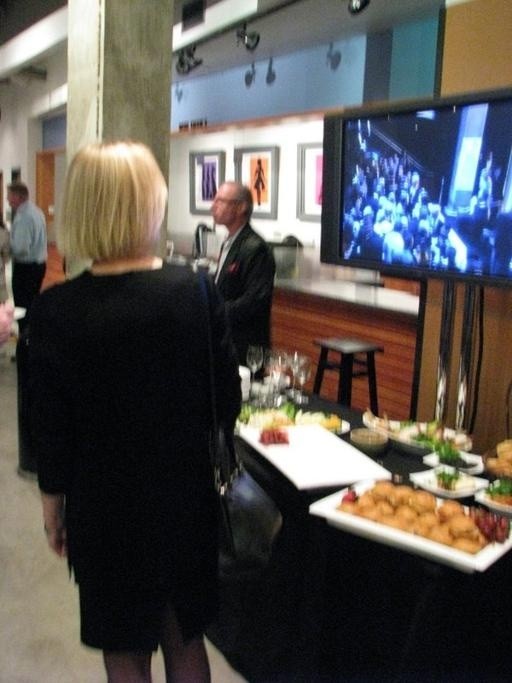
[319,86,512,286]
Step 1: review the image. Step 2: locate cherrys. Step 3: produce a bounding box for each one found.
[476,510,512,544]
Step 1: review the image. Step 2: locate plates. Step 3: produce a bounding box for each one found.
[421,448,485,476]
[363,412,472,455]
[238,422,398,493]
[234,411,351,434]
[475,475,511,513]
[305,481,511,575]
[409,465,493,498]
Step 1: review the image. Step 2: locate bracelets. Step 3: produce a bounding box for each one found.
[45,522,51,534]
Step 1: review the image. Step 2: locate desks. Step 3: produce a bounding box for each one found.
[202,379,512,682]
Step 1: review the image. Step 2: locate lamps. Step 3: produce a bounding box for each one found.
[346,0,371,15]
[233,23,260,51]
[173,41,205,75]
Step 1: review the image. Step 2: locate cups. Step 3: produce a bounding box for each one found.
[167,240,174,258]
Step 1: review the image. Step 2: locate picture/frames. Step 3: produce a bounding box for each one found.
[296,138,325,227]
[231,142,282,221]
[187,147,227,217]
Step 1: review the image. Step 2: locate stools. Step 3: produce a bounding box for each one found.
[309,334,383,414]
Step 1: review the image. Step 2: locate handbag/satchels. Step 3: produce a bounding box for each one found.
[215,448,282,588]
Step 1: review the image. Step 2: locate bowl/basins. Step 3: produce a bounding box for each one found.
[349,428,387,454]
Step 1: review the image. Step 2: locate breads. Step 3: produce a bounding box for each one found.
[486,438,511,479]
[344,480,487,555]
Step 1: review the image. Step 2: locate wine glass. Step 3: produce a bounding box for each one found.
[242,340,313,408]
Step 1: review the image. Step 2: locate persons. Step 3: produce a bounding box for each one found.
[0,213,13,304]
[342,145,512,278]
[211,182,276,383]
[7,183,47,334]
[15,139,242,682]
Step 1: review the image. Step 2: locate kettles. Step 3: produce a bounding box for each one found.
[194,224,208,259]
